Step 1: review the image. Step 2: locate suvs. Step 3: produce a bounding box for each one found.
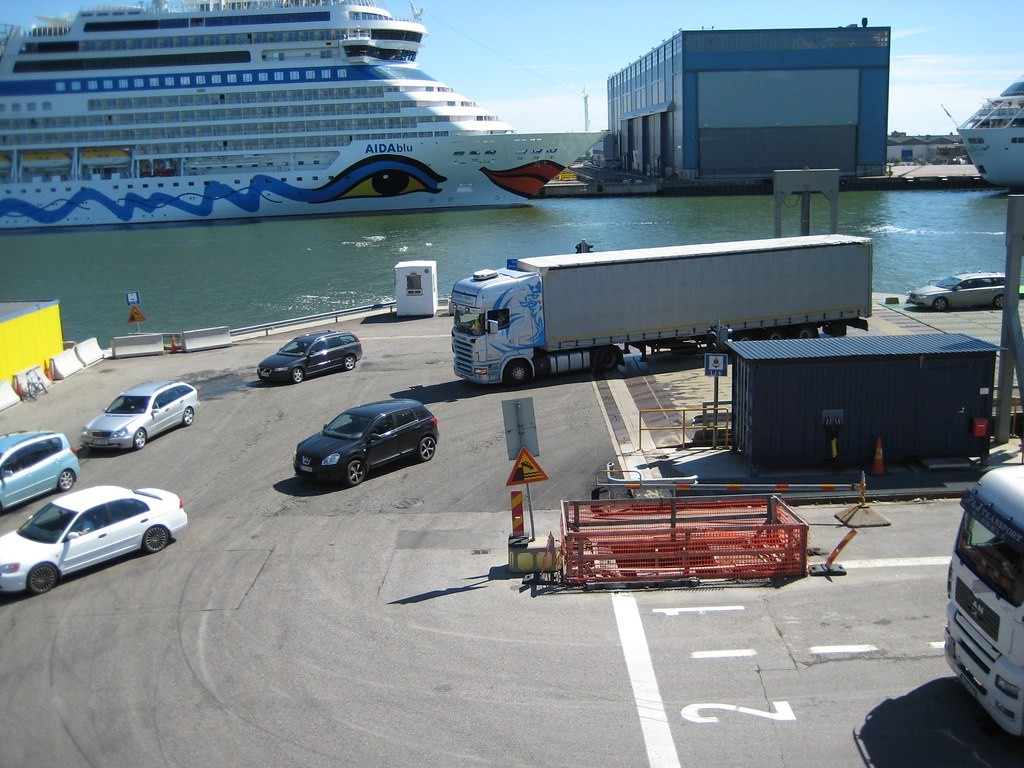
[293,399,438,487]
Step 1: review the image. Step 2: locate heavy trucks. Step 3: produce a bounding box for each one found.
[452,234,874,390]
[945,465,1024,735]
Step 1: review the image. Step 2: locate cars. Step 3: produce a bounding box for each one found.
[0,486,188,593]
[0,430,80,517]
[953,155,966,164]
[257,330,362,386]
[81,380,200,449]
[909,272,1005,313]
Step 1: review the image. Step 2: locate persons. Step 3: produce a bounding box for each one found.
[152,399,159,409]
[496,310,507,329]
[73,515,96,533]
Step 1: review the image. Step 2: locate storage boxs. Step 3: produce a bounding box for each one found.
[972,417,989,438]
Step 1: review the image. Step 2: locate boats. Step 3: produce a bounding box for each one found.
[957,75,1024,184]
[0,0,610,229]
